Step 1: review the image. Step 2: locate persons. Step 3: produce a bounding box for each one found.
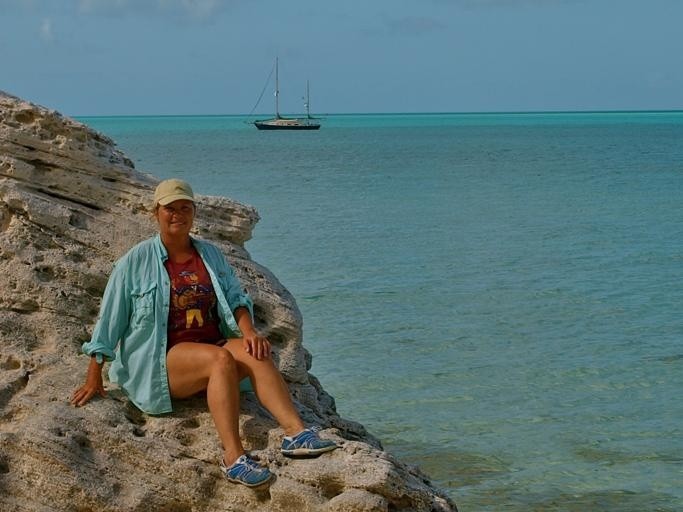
[70,178,337,488]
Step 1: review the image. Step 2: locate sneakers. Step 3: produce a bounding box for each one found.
[219,453,273,487]
[281,429,337,456]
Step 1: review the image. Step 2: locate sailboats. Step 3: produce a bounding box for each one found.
[243,56,327,131]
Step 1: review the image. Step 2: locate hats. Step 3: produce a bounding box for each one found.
[153,178,195,209]
